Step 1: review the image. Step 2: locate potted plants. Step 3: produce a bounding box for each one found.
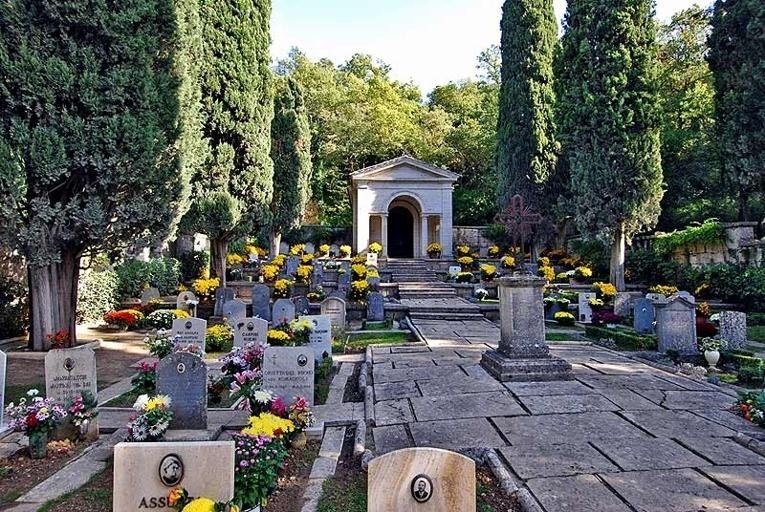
[699,336,727,368]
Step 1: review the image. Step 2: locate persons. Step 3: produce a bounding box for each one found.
[247,323,253,330]
[414,480,428,498]
[178,364,183,371]
[162,459,180,484]
[299,356,305,364]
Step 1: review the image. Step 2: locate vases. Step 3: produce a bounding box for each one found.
[288,431,306,449]
[29,429,48,458]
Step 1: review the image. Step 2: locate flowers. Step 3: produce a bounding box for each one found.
[290,395,315,430]
[6,389,67,443]
